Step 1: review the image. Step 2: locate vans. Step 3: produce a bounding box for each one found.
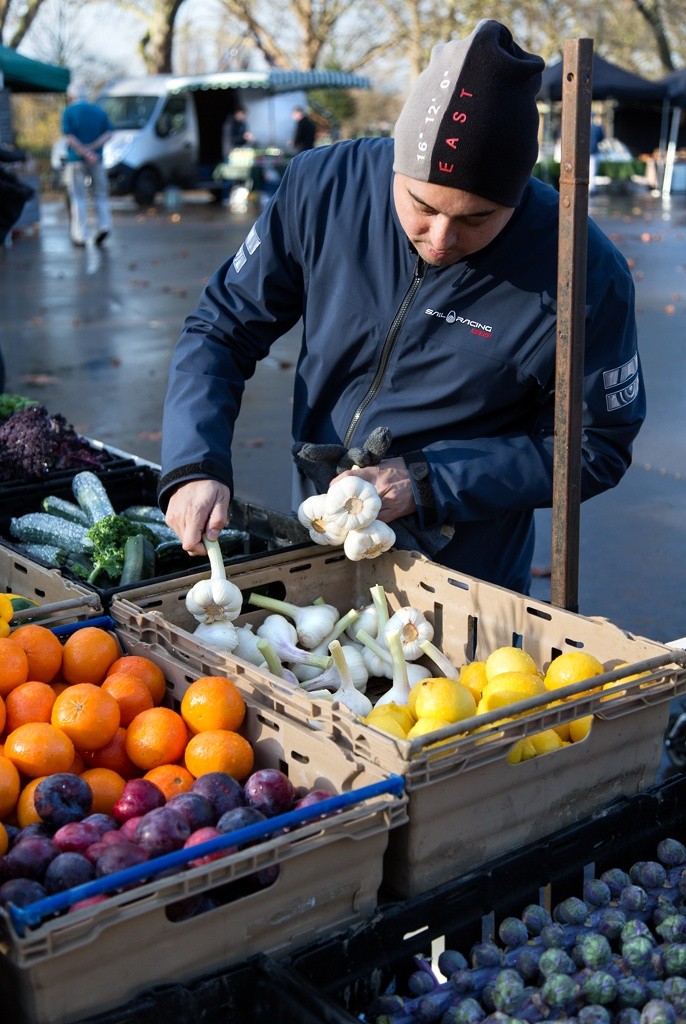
[50,74,311,209]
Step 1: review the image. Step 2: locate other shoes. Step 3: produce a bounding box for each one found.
[69,239,86,247]
[95,232,109,245]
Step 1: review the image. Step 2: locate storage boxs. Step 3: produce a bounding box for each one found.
[0,426,686,1024]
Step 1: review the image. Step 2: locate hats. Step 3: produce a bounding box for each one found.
[67,79,89,99]
[391,19,546,207]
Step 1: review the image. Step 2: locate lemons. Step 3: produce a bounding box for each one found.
[361,648,659,765]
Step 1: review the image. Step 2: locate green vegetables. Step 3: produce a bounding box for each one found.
[0,393,96,481]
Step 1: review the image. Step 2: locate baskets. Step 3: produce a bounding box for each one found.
[0,409,686,1024]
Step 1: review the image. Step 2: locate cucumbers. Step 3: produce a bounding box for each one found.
[9,471,249,582]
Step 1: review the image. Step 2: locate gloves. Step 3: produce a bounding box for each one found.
[292,427,456,560]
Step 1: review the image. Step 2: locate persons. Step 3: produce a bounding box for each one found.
[62,82,115,246]
[156,20,647,657]
[220,106,255,208]
[290,107,317,153]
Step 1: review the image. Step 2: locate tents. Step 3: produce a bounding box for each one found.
[535,53,686,211]
[0,46,68,94]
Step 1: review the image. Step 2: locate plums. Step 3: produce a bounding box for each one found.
[0,767,354,942]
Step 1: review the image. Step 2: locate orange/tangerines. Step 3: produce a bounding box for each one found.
[0,624,254,854]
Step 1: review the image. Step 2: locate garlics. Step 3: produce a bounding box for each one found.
[185,474,464,715]
